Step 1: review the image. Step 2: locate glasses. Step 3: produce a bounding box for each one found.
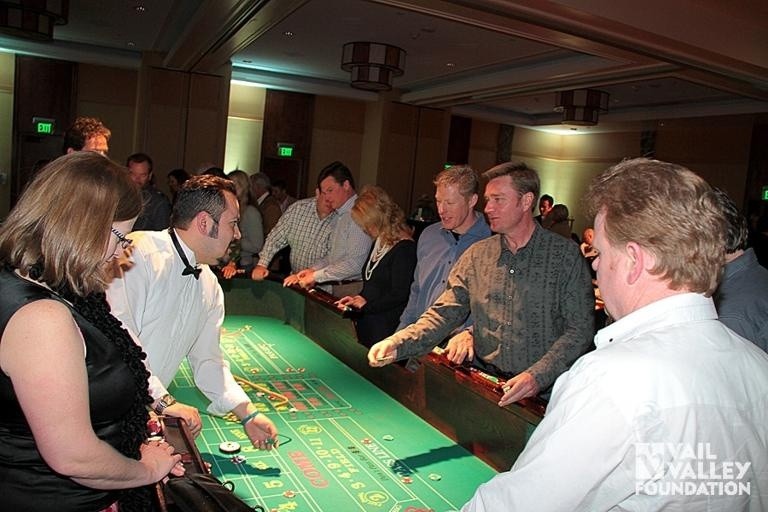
[109,226,134,252]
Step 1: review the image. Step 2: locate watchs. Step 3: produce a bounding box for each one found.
[154,393,176,412]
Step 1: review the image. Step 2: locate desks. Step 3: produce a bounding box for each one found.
[142,267,546,511]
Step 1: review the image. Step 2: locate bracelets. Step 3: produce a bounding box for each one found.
[240,409,259,423]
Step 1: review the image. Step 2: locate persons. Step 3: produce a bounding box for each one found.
[395,165,492,335]
[272,182,296,208]
[0,151,184,512]
[252,185,333,288]
[368,163,596,407]
[336,186,416,344]
[65,118,110,155]
[225,171,262,270]
[453,158,768,511]
[705,187,767,352]
[128,152,172,230]
[297,160,374,300]
[106,174,280,452]
[547,204,574,235]
[538,195,553,225]
[251,172,283,273]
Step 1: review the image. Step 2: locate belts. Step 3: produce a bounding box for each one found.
[329,278,364,286]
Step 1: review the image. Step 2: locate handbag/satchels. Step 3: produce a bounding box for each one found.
[166,472,264,512]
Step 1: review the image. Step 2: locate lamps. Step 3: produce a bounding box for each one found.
[127,39,136,48]
[280,28,295,40]
[340,41,408,92]
[553,89,610,127]
[134,4,147,16]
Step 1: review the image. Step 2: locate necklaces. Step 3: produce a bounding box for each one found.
[365,234,389,282]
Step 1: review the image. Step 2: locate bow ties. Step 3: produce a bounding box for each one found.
[167,227,204,281]
